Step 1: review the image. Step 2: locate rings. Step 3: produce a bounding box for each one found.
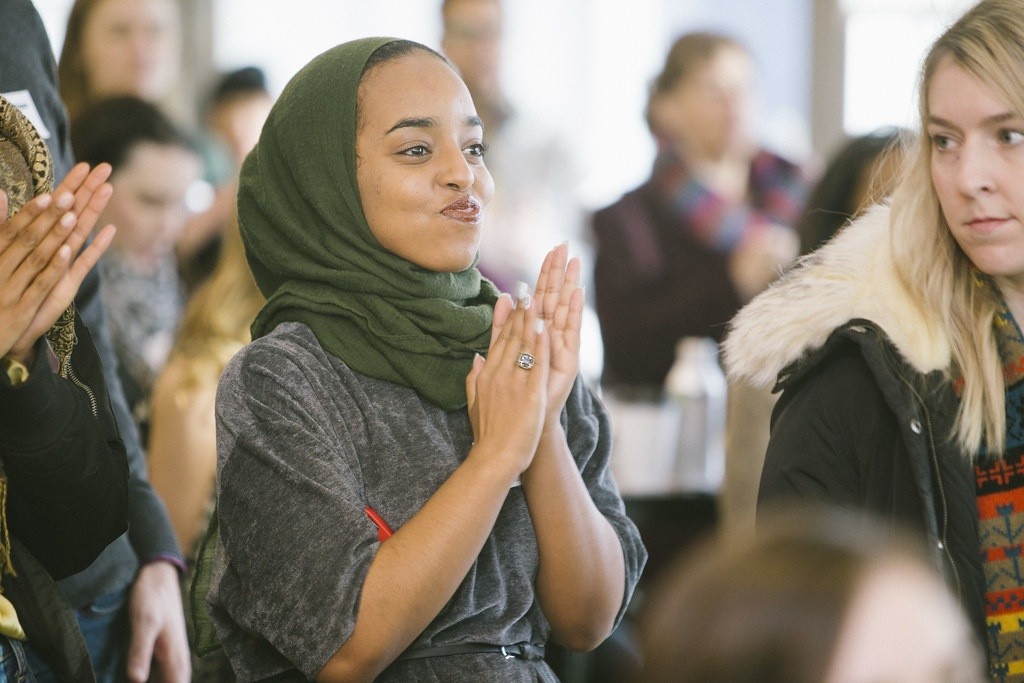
[520,353,534,369]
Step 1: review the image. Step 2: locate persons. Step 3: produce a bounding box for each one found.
[185,35,646,683]
[436,0,597,304]
[592,30,820,405]
[57,0,274,569]
[648,506,989,683]
[800,123,909,254]
[721,0,1024,683]
[0,0,194,683]
